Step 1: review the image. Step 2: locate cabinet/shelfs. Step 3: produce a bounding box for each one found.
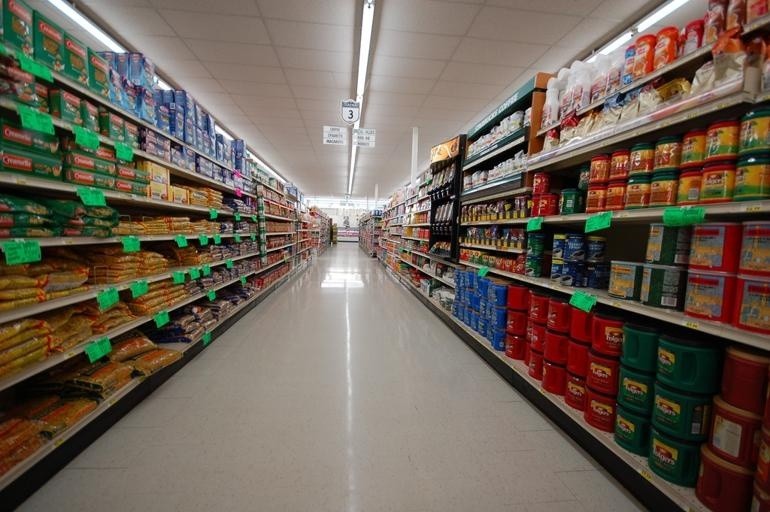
[360,15,770,511]
[0,40,337,492]
